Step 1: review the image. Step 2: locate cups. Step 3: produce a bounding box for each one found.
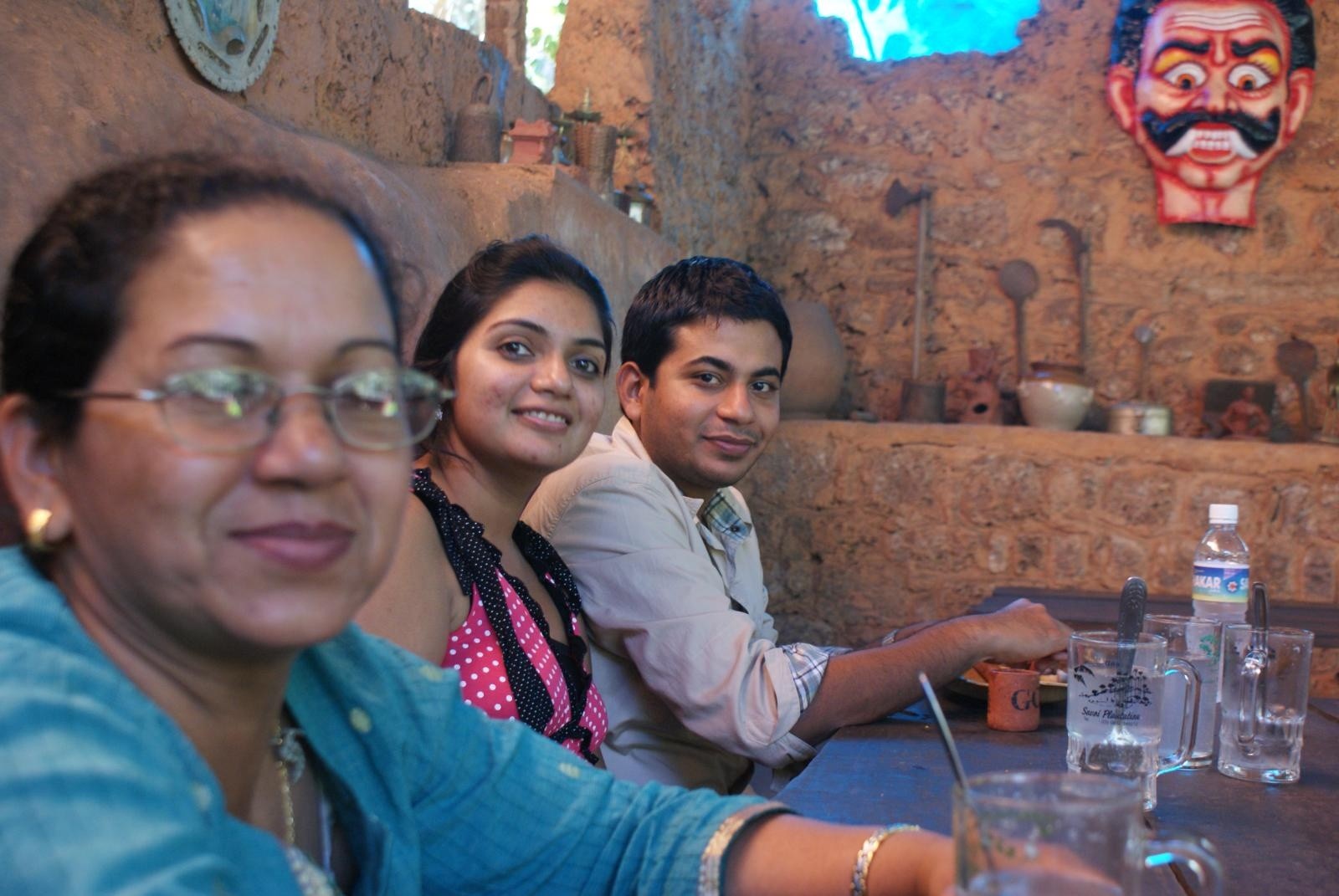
[952,771,1225,896]
[1141,614,1224,766]
[1067,631,1200,814]
[1217,623,1314,785]
[988,668,1041,732]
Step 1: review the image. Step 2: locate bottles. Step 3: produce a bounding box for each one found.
[1191,503,1250,705]
[1016,361,1100,430]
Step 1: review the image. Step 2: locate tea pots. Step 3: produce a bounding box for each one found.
[448,73,502,162]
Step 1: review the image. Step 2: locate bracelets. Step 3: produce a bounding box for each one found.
[849,822,921,896]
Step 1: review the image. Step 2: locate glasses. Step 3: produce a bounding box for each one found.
[28,364,457,452]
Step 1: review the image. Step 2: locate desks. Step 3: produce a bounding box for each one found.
[766,579,1339,896]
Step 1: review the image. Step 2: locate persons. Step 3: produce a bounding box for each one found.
[521,256,1075,798]
[1,149,1124,895]
[353,234,619,776]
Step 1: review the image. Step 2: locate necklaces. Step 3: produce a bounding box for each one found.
[275,725,298,847]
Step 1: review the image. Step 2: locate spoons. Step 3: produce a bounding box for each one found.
[1251,581,1283,751]
[1079,577,1149,781]
